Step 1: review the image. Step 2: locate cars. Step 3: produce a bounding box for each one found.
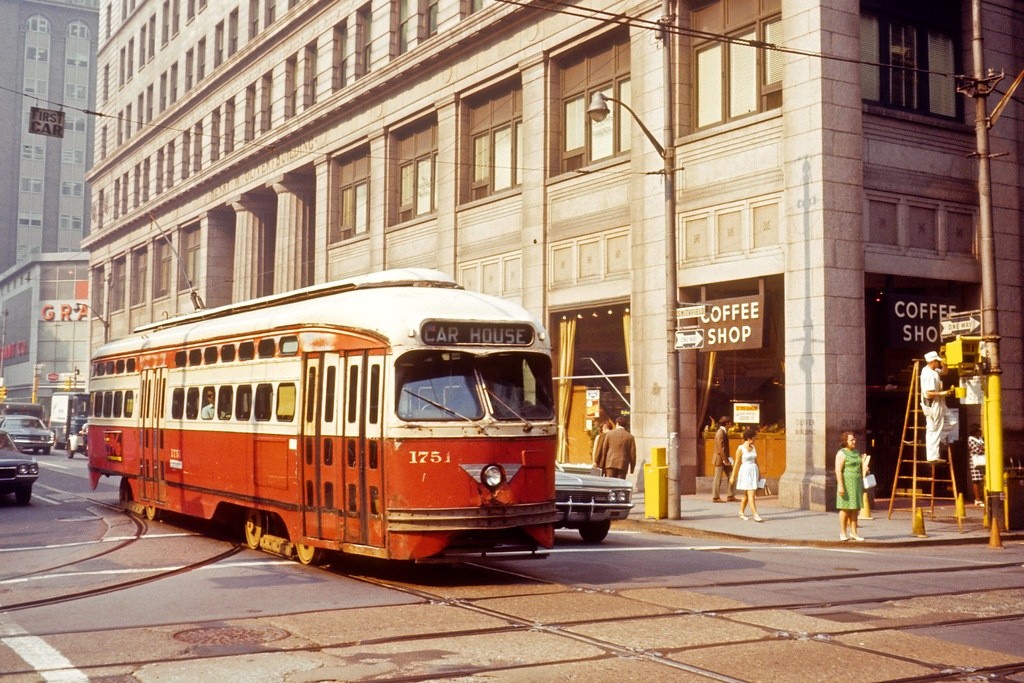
[0,415,55,455]
[549,460,635,541]
[0,430,40,504]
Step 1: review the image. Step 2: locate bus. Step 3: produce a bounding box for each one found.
[86,267,558,568]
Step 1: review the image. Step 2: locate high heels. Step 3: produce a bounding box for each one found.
[850,533,865,541]
[840,534,848,541]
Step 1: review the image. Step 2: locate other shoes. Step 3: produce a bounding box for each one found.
[975,500,984,507]
[753,512,763,521]
[713,498,727,503]
[739,512,748,520]
[927,458,947,463]
[727,497,742,502]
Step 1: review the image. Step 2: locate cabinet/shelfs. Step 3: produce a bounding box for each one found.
[567,385,630,465]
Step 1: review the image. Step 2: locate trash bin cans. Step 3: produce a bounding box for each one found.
[644,447,669,520]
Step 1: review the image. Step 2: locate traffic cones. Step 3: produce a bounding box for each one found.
[909,508,928,538]
[986,518,1005,549]
[951,492,971,518]
[857,492,875,520]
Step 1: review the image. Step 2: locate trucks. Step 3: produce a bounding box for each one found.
[49,391,89,448]
[0,402,43,432]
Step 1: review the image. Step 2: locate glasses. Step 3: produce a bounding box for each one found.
[208,394,215,398]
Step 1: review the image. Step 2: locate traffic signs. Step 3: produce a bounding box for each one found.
[674,329,705,349]
[938,315,980,336]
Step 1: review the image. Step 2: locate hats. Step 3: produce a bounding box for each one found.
[924,351,942,362]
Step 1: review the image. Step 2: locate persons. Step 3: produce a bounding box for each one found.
[920,350,955,464]
[713,416,741,503]
[593,417,636,479]
[729,430,762,522]
[967,422,985,507]
[835,431,865,542]
[201,387,231,420]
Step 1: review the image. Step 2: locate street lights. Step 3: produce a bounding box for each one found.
[585,89,681,522]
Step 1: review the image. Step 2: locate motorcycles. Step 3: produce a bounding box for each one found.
[66,434,89,459]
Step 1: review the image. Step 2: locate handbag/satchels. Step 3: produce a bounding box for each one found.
[756,484,771,497]
[863,474,877,489]
[972,454,985,467]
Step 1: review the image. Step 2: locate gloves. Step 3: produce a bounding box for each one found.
[940,346,946,358]
[947,385,955,396]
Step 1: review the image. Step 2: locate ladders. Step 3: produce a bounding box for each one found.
[888,358,965,534]
